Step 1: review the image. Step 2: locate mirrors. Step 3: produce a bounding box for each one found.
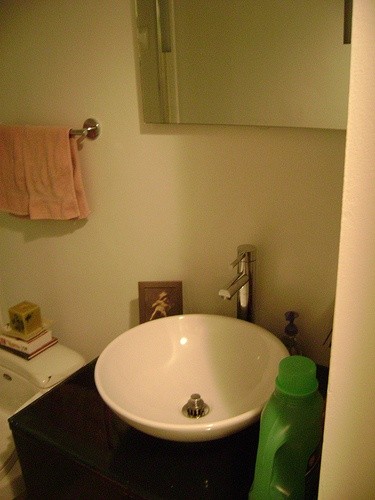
[132,0,353,130]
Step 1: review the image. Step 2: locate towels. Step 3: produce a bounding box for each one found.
[0,123,90,222]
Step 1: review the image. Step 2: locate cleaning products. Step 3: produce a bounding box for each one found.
[246,355,324,500]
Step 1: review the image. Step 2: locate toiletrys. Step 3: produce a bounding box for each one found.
[274,310,307,356]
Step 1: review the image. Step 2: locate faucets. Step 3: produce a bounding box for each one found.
[217,243,257,323]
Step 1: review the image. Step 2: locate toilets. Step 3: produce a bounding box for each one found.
[0,330,87,500]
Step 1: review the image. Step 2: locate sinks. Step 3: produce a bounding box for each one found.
[93,313,291,442]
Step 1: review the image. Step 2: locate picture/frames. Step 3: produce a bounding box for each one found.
[138,281,184,325]
